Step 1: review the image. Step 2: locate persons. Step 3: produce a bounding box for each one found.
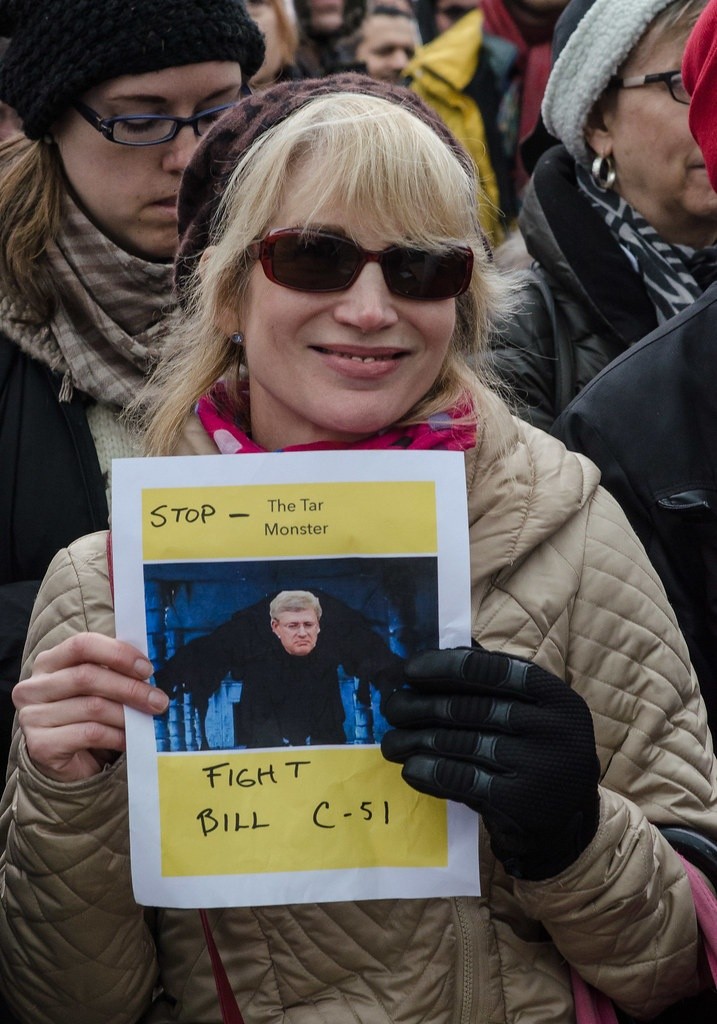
[0,72,717,1024]
[269,590,322,656]
[1,1,717,789]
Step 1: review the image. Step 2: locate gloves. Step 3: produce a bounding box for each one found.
[381,637,601,881]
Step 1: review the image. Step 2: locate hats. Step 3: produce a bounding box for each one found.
[540,1,677,175]
[681,0,717,194]
[174,70,495,316]
[0,0,267,141]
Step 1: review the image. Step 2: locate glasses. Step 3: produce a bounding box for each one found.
[616,69,691,105]
[241,225,474,302]
[70,99,240,147]
